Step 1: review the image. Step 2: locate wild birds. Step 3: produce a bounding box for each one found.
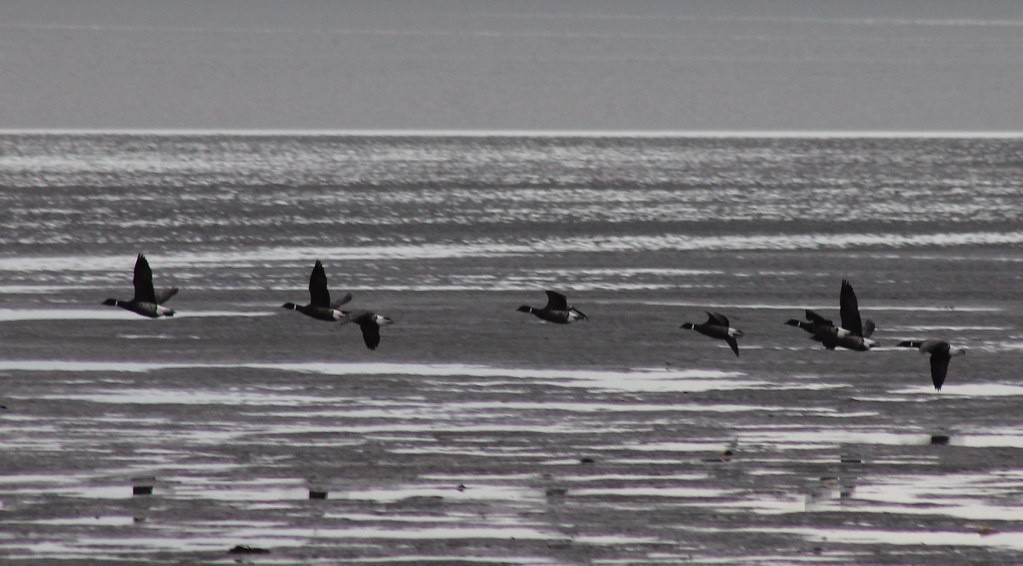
[894,338,967,395]
[678,310,747,363]
[281,258,394,351]
[784,279,881,352]
[102,250,179,319]
[514,290,589,326]
